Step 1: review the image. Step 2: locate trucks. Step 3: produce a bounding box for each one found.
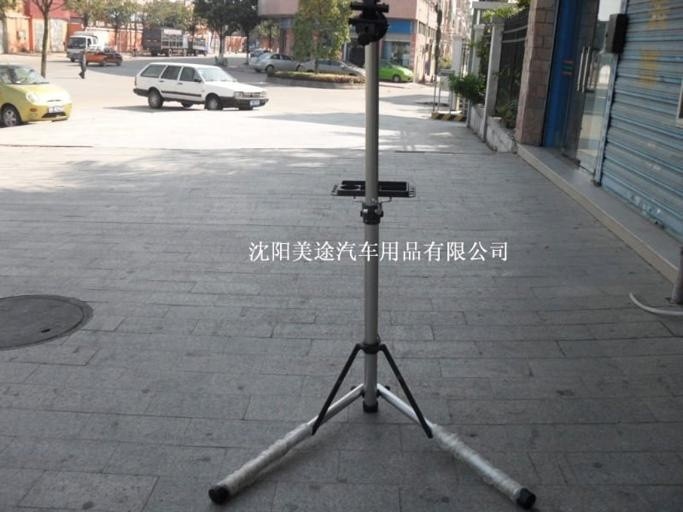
[143,27,208,57]
[66,31,108,62]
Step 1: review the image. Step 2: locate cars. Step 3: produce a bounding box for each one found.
[0,64,73,127]
[248,49,414,83]
[80,48,122,66]
[132,62,269,110]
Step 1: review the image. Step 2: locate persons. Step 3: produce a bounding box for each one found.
[79,48,87,79]
